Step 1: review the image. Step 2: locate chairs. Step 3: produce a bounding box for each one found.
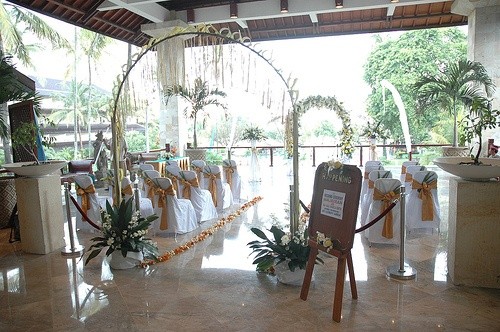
[204,166,231,210]
[405,171,440,234]
[150,178,197,235]
[222,160,246,201]
[177,170,216,226]
[364,179,403,248]
[361,170,391,220]
[403,166,428,188]
[165,167,183,197]
[165,161,179,169]
[400,162,420,184]
[190,160,207,188]
[143,170,160,195]
[74,176,111,232]
[365,161,381,166]
[138,163,154,186]
[111,178,155,227]
[362,165,385,198]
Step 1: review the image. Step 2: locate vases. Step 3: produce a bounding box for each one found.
[273,260,316,285]
[108,249,144,270]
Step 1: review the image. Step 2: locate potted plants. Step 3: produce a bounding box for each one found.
[0,120,70,177]
[162,77,229,162]
[431,96,499,181]
[413,59,496,158]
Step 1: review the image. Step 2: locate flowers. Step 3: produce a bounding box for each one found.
[138,196,262,269]
[248,201,339,272]
[77,196,160,267]
[282,96,354,171]
